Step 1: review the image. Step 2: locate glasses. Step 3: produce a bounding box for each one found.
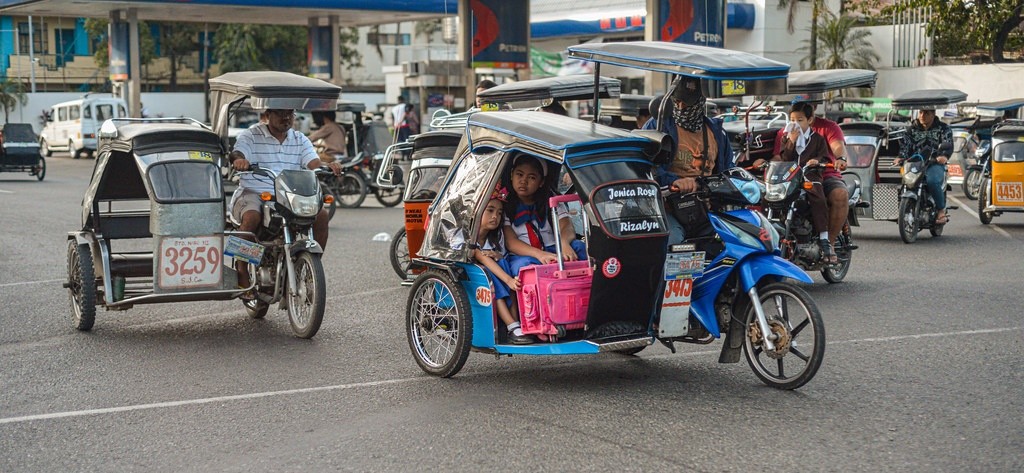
[271,111,296,119]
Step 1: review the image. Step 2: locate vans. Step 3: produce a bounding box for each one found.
[38,97,127,159]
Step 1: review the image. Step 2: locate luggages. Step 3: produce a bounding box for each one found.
[516,194,594,341]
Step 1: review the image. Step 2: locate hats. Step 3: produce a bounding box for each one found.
[675,76,703,106]
[513,152,547,176]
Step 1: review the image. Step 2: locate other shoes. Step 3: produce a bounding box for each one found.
[506,327,533,344]
[820,240,832,255]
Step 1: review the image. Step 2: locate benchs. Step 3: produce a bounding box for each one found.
[877,167,903,184]
[95,216,153,276]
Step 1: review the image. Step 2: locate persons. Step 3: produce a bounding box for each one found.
[451,152,604,347]
[770,96,849,265]
[465,80,506,109]
[542,98,567,116]
[226,109,348,299]
[634,109,655,128]
[641,73,738,260]
[712,101,744,122]
[387,94,419,163]
[891,109,954,237]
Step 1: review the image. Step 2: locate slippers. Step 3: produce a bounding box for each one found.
[233,285,255,299]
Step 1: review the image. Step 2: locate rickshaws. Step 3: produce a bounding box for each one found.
[406,43,846,391]
[837,89,970,243]
[0,123,46,180]
[61,70,350,342]
[946,98,1023,226]
[734,70,877,283]
[337,72,873,212]
[778,67,877,126]
[207,72,346,222]
[842,88,985,245]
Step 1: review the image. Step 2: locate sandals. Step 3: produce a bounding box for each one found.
[935,210,948,224]
[824,245,838,261]
